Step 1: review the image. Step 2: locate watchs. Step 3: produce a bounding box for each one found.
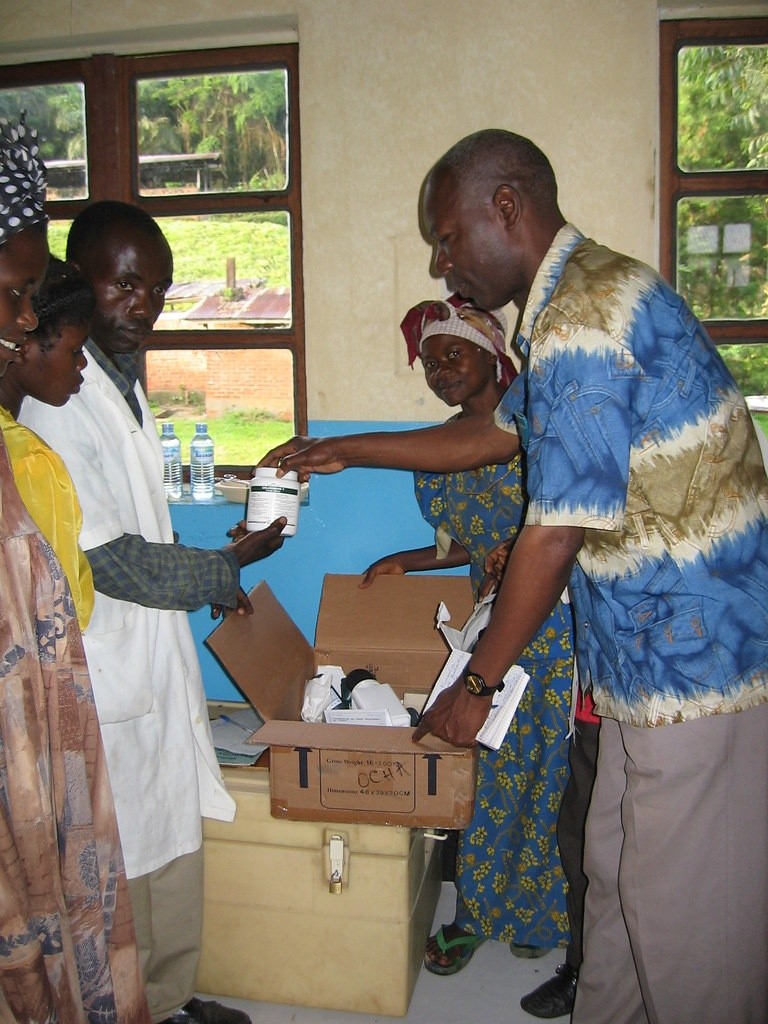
[463,661,505,697]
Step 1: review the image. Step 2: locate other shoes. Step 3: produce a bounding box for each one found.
[519,964,577,1018]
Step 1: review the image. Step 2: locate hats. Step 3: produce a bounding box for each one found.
[399,291,520,390]
[1,110,50,241]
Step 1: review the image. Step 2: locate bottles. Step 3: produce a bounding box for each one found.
[159,423,183,502]
[190,423,214,501]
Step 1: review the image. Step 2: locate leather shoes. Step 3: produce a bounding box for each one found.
[161,997,251,1024]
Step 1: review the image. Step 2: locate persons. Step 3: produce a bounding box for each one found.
[359,294,577,975]
[0,119,287,1024]
[253,128,768,1024]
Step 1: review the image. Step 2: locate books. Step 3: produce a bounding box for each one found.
[422,649,530,751]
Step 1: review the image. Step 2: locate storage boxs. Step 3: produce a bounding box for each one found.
[203,574,479,831]
[196,699,445,1018]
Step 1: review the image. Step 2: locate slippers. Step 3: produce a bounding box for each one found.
[509,941,553,958]
[424,923,489,976]
